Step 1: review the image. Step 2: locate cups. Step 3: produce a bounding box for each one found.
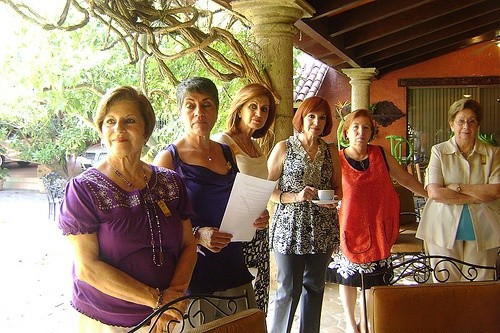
[314,189,334,200]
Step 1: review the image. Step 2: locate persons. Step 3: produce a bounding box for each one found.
[267,97,344,333]
[325,109,430,333]
[58,84,200,333]
[416,99,500,283]
[212,84,270,314]
[152,77,270,333]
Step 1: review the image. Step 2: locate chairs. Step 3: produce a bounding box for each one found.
[39,172,67,221]
[360,254,500,333]
[127,289,267,333]
[390,184,432,279]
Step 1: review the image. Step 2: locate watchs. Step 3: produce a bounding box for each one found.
[457,183,461,193]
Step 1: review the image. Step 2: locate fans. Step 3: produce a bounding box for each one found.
[446,29,500,58]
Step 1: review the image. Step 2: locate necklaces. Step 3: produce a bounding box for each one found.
[232,129,254,158]
[300,138,315,152]
[460,141,474,156]
[349,147,365,170]
[184,136,211,161]
[107,160,163,266]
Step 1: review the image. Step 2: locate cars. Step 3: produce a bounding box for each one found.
[70,144,107,170]
[1,118,46,168]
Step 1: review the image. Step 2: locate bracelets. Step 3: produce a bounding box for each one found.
[155,287,164,310]
[278,191,284,205]
[194,226,199,235]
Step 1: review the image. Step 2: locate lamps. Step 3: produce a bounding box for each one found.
[495,28,500,48]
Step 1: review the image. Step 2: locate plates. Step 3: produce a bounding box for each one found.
[313,200,339,204]
